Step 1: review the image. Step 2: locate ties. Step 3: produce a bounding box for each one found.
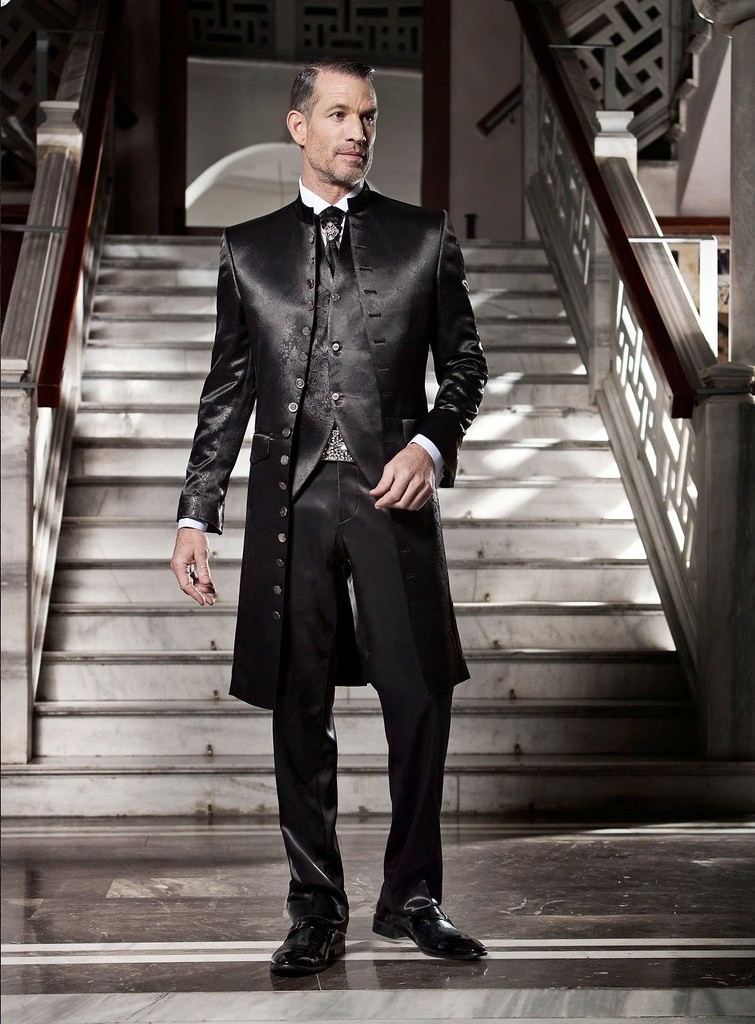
[320,204,346,274]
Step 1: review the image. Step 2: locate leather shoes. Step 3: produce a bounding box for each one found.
[270,920,345,975]
[372,904,488,961]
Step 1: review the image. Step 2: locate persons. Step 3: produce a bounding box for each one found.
[171,58,488,975]
